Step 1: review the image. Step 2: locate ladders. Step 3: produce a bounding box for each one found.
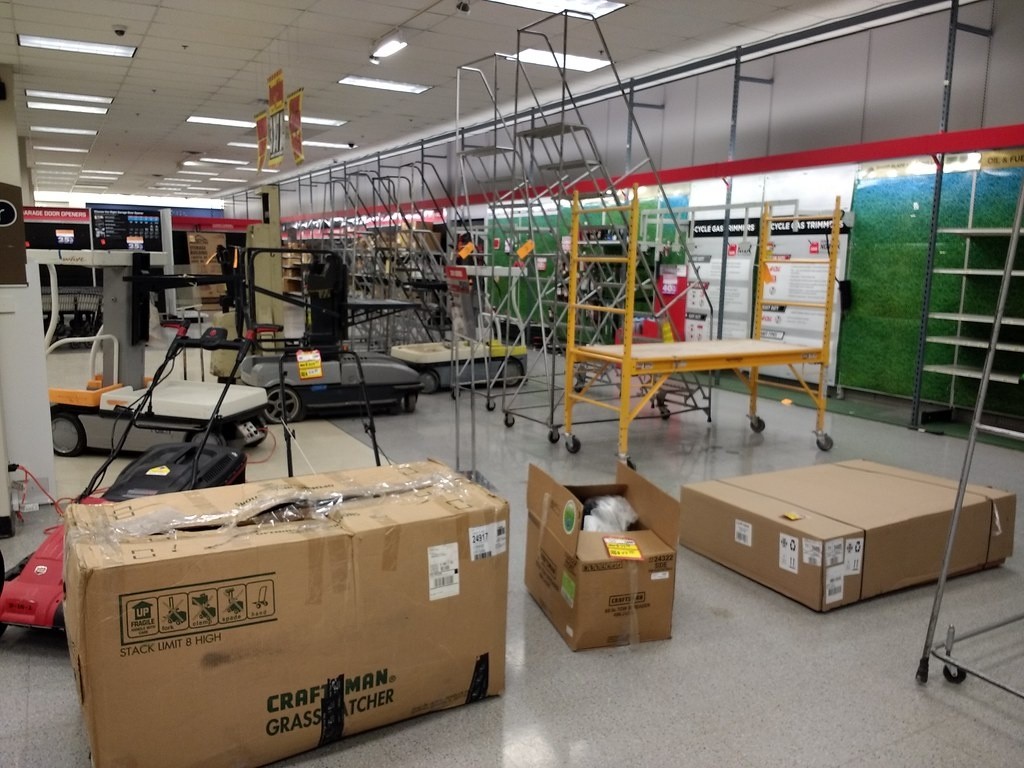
[323,162,502,358]
[450,54,666,411]
[500,10,713,453]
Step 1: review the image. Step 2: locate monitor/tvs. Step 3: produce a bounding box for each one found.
[23,207,162,251]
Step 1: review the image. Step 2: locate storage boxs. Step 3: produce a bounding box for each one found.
[677,459,1017,613]
[58,459,513,767]
[522,461,680,653]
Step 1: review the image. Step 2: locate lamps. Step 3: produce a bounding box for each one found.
[373,27,407,58]
